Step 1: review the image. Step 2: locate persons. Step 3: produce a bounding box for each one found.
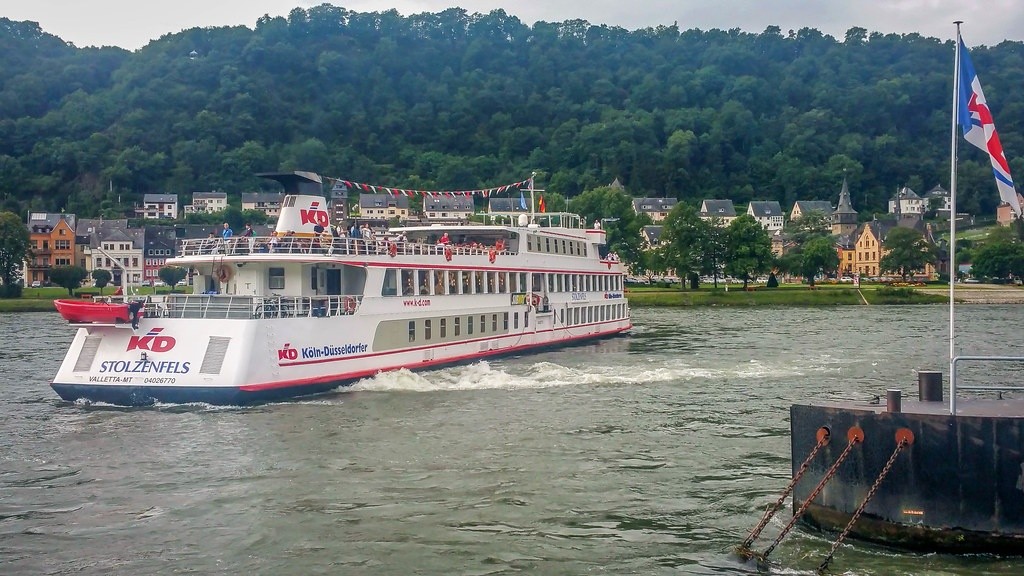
[600,252,620,261]
[531,219,536,224]
[543,294,549,313]
[202,219,506,254]
[532,292,540,313]
[594,220,600,229]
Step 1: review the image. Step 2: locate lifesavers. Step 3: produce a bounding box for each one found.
[216,265,230,282]
[344,296,355,312]
[390,243,397,257]
[489,251,496,263]
[446,249,452,261]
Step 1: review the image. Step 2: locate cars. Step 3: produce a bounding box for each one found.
[32,280,44,289]
[154,279,166,287]
[804,277,930,284]
[142,280,150,287]
[623,274,776,285]
[176,279,187,286]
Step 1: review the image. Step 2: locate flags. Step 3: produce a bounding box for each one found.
[520,191,529,210]
[538,193,547,213]
[952,31,1022,219]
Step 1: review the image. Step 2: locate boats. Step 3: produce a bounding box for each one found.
[53,298,147,323]
[48,195,635,409]
